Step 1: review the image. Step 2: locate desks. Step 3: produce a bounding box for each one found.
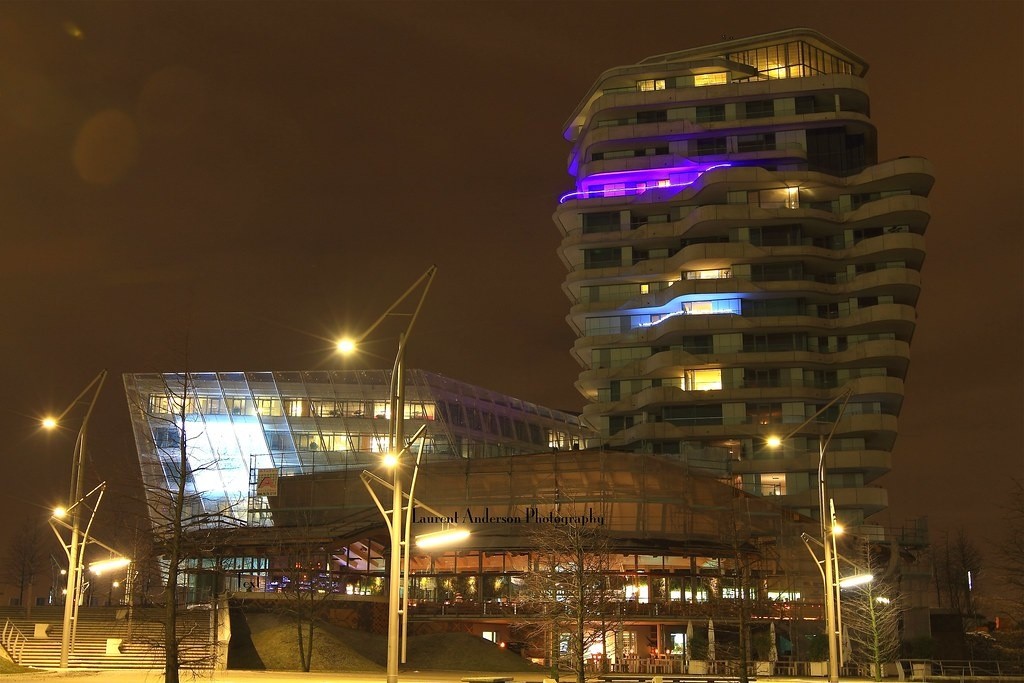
[461,676,514,683]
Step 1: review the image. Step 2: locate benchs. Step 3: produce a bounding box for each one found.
[597,675,758,683]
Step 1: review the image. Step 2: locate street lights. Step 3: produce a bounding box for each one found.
[336,267,466,683]
[771,383,874,683]
[44,367,109,676]
